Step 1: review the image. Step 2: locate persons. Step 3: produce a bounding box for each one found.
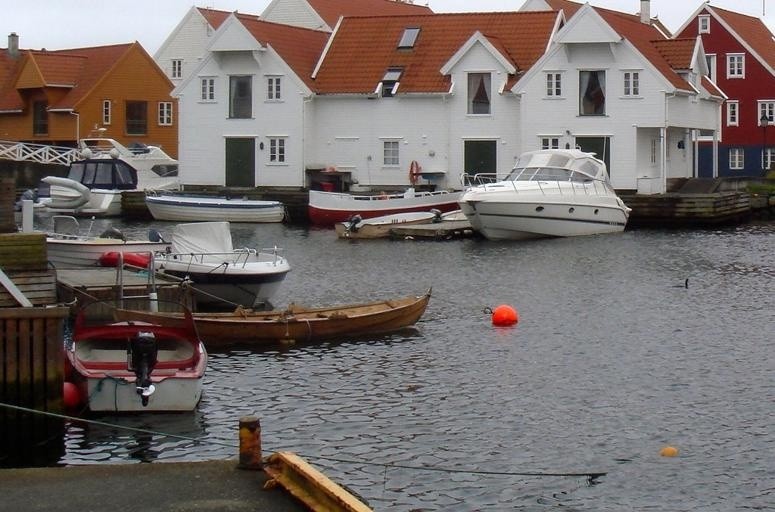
[127,330,159,406]
[127,420,159,462]
[17,185,39,206]
